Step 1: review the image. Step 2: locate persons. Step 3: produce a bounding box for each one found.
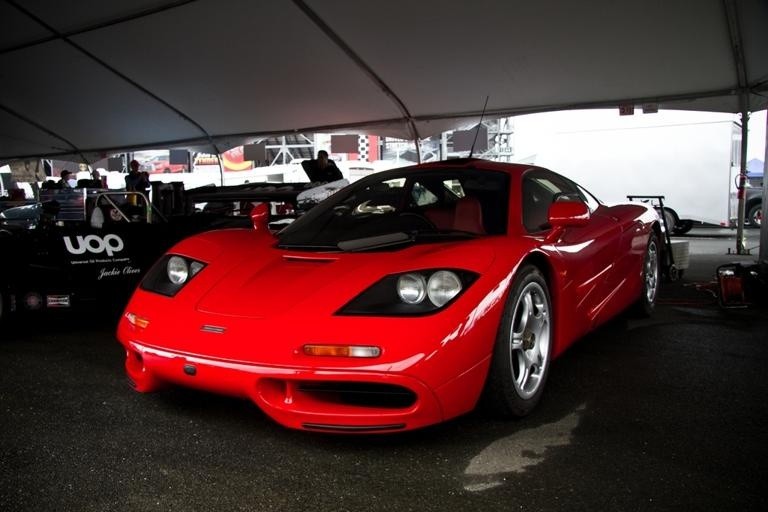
[57,170,73,189]
[86,170,109,189]
[124,159,150,193]
[307,149,344,183]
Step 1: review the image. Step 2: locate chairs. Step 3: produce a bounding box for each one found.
[453,199,484,236]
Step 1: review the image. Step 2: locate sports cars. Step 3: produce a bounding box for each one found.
[117,96,667,434]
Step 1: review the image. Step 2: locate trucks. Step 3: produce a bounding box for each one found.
[498,110,749,233]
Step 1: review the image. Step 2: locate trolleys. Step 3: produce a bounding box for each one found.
[626,195,681,282]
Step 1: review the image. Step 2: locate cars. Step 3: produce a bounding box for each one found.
[0,159,412,329]
[139,156,188,174]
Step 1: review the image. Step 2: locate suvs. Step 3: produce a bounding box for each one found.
[745,177,763,231]
